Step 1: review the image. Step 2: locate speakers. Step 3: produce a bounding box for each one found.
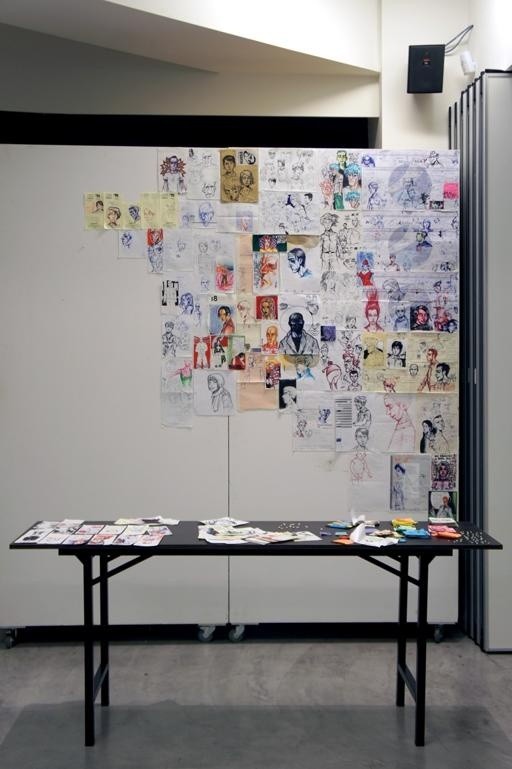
[460,51,475,75]
[407,44,445,94]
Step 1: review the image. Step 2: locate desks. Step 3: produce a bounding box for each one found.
[7,520,504,747]
